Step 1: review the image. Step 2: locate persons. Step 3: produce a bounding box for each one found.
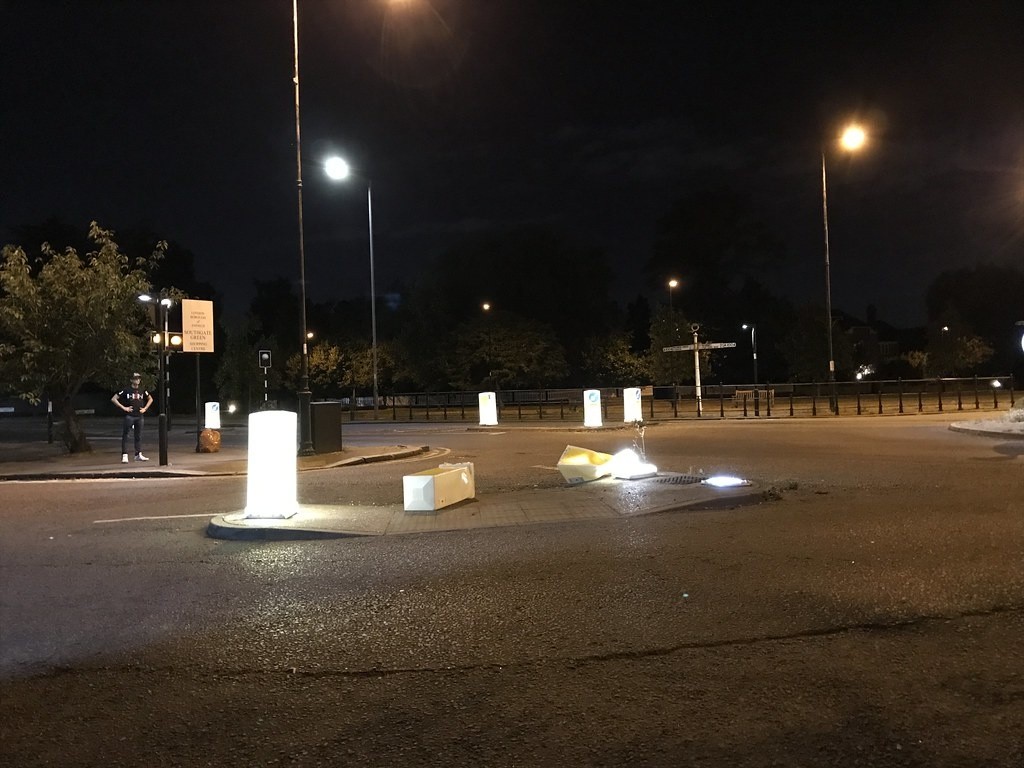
[111,373,154,464]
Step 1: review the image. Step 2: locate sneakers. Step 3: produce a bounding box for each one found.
[122,454,128,463]
[134,452,149,461]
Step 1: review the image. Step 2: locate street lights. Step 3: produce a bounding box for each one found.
[667,278,681,386]
[481,301,496,391]
[293,0,439,453]
[324,152,381,421]
[821,123,866,411]
[940,326,949,345]
[138,289,172,466]
[742,322,759,417]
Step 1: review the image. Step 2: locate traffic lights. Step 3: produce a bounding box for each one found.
[150,330,183,352]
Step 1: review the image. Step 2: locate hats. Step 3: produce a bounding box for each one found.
[130,373,142,378]
